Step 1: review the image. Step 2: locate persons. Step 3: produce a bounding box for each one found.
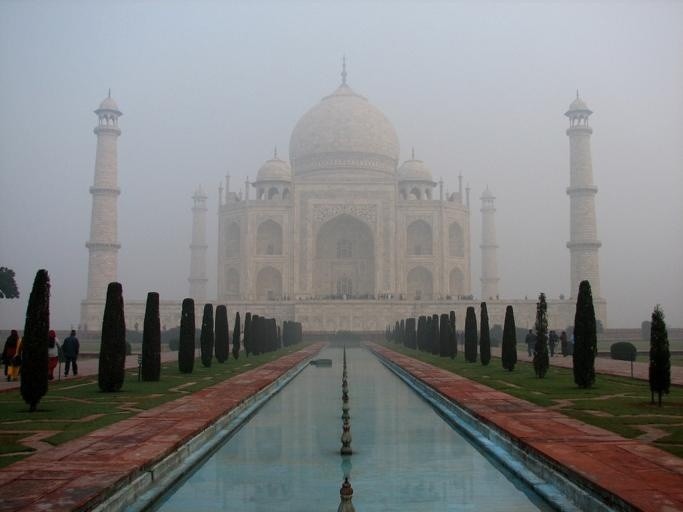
[61,328,81,379]
[524,328,535,360]
[552,331,559,354]
[1,329,22,383]
[547,330,555,357]
[558,330,568,357]
[48,328,61,383]
